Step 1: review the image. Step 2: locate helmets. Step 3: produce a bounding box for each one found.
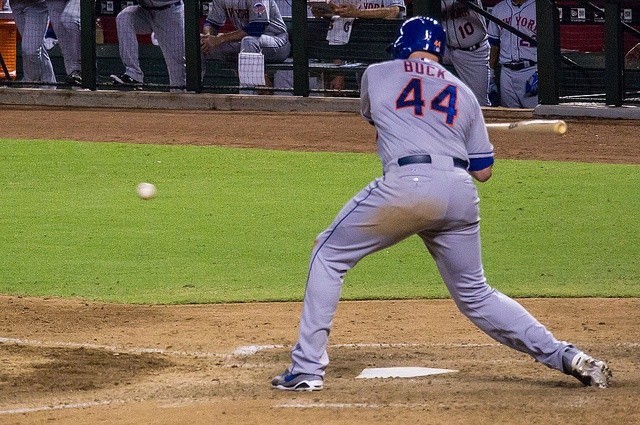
[385,16,446,65]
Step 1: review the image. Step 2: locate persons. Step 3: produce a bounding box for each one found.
[488,0,540,108]
[46,0,81,79]
[0,0,56,90]
[417,0,492,107]
[309,1,405,19]
[110,1,187,93]
[271,16,613,390]
[202,0,290,95]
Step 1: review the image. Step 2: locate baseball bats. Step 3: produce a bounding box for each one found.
[485,120,567,135]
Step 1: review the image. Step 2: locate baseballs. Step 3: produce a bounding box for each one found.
[136,183,155,199]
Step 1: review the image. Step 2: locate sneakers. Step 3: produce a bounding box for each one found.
[563,348,613,389]
[271,370,323,393]
[109,73,143,89]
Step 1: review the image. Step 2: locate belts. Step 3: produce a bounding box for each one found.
[502,60,535,71]
[396,154,468,170]
[448,45,483,51]
[143,0,180,10]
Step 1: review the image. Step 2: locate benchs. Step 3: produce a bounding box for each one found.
[205,15,405,96]
